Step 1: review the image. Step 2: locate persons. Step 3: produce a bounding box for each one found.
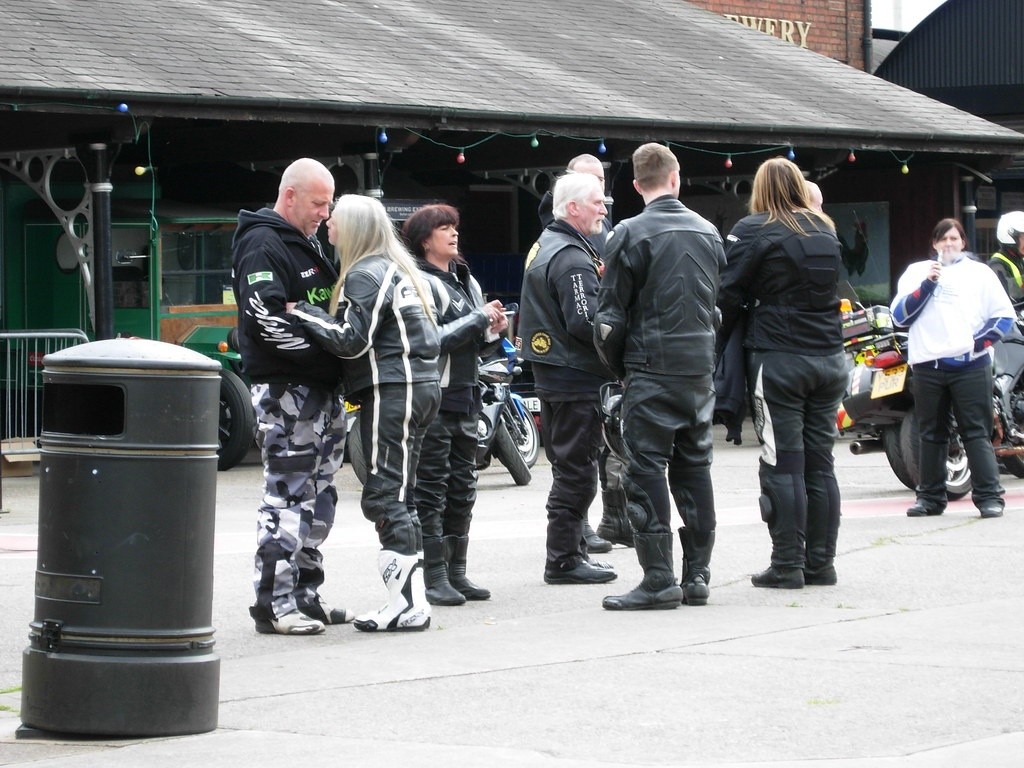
[989,211,1024,319]
[537,154,636,554]
[516,172,618,586]
[806,181,823,212]
[288,193,440,633]
[401,205,508,603]
[232,157,355,634]
[718,156,850,587]
[888,219,1015,519]
[595,142,729,611]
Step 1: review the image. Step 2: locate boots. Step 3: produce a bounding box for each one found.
[597,490,636,547]
[602,531,683,610]
[354,549,431,631]
[678,527,715,607]
[443,535,491,600]
[421,537,466,606]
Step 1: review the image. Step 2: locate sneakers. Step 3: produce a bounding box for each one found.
[255,605,326,635]
[295,600,354,623]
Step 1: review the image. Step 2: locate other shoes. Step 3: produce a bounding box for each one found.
[804,564,838,585]
[980,505,1003,516]
[906,498,929,516]
[584,557,614,570]
[543,558,617,585]
[751,564,805,590]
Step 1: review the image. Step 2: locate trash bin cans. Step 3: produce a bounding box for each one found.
[13,338,219,742]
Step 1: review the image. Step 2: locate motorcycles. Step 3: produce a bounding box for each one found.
[344,302,540,486]
[837,299,1023,500]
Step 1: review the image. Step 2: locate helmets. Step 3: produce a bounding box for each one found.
[997,211,1024,244]
